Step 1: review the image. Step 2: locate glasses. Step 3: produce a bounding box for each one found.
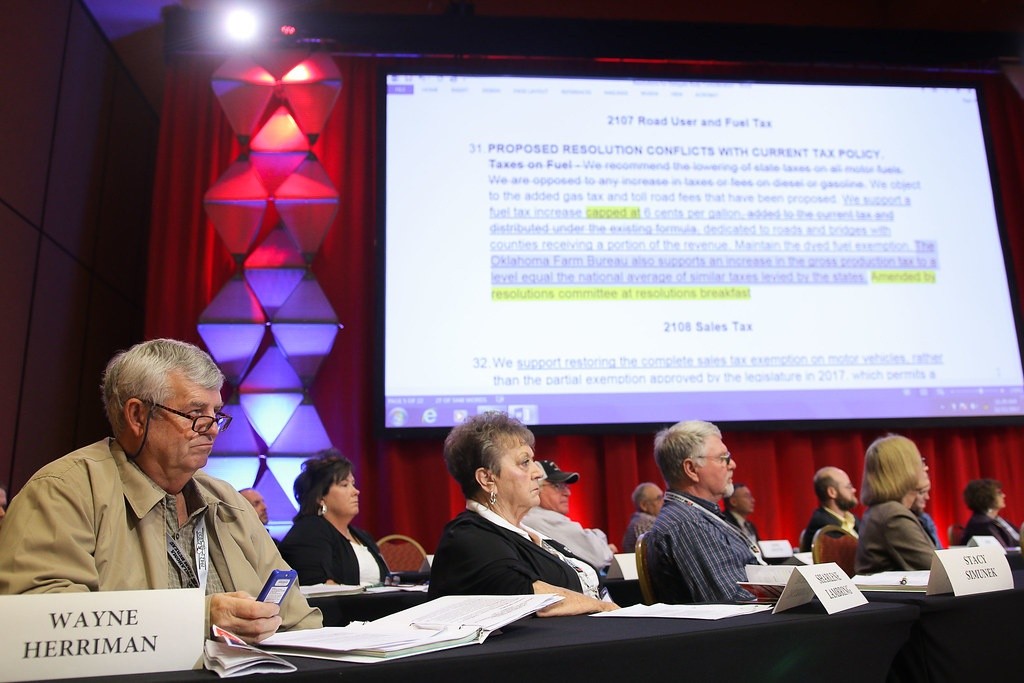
[141,400,233,431]
[538,481,565,490]
[691,452,730,466]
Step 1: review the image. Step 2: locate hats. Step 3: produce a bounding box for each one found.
[533,460,579,483]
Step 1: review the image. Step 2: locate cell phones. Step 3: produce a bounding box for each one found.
[256,569,296,605]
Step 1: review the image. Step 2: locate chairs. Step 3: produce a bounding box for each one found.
[375,535,430,573]
[635,532,656,606]
[949,524,966,547]
[812,525,859,580]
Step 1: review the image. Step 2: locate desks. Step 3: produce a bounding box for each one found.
[8,552,1024,683]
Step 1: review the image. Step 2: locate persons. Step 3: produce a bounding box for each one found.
[800,466,861,552]
[239,488,269,525]
[854,435,936,576]
[278,449,390,587]
[0,338,324,644]
[425,411,621,617]
[909,478,942,549]
[520,461,619,574]
[624,483,663,553]
[723,484,760,541]
[0,486,7,523]
[963,478,1020,547]
[646,419,769,603]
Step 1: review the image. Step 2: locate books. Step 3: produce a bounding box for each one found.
[257,593,566,665]
[851,571,931,592]
